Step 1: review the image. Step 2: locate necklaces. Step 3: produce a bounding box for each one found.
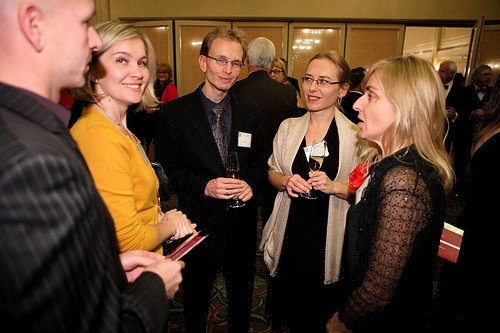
[104,108,149,165]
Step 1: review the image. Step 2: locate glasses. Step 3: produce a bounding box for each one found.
[476,73,493,76]
[269,69,283,75]
[301,76,342,88]
[204,54,243,69]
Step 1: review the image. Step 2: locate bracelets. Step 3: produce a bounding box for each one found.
[162,220,176,236]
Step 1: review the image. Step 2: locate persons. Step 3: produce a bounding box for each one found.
[232,37,307,228]
[260,50,373,333]
[69,20,197,256]
[155,24,273,333]
[0,0,183,333]
[437,61,500,333]
[339,67,367,125]
[326,57,455,333]
[153,63,177,105]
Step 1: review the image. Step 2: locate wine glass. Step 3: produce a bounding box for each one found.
[299,138,328,200]
[225,152,245,208]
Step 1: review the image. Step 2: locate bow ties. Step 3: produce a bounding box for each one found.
[476,87,485,94]
[443,84,448,90]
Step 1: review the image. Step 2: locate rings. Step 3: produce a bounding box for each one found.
[316,181,319,187]
[291,185,295,189]
[226,190,228,195]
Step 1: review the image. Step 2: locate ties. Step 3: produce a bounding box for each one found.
[212,105,228,152]
